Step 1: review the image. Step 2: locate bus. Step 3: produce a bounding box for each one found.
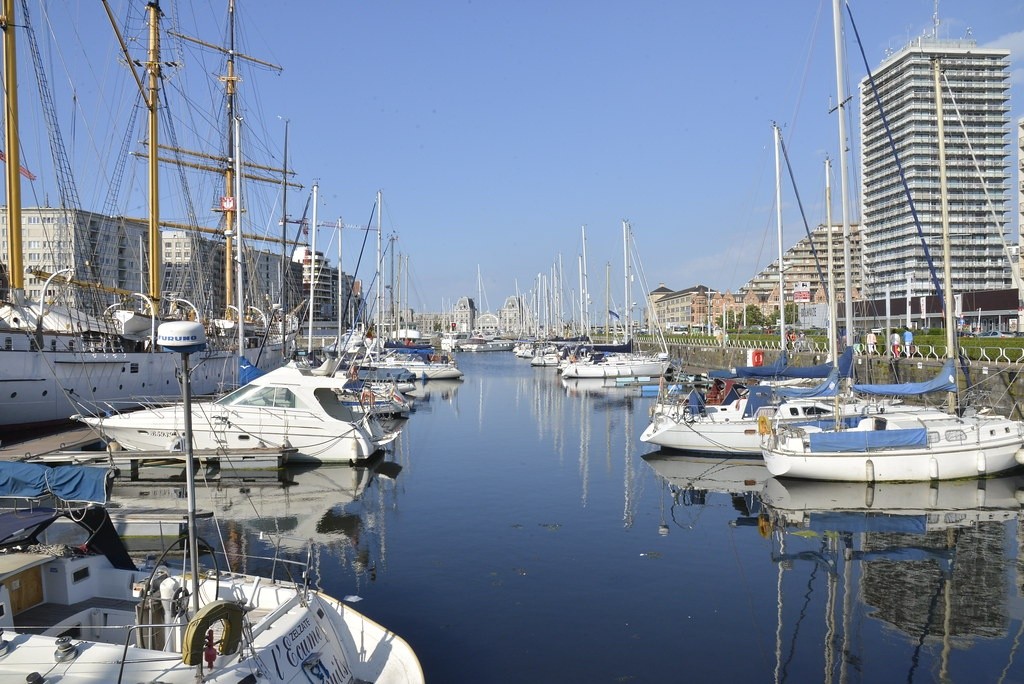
[668,325,689,337]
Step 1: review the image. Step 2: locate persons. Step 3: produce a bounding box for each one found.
[901,327,914,357]
[866,330,877,355]
[716,328,730,347]
[785,329,808,352]
[890,329,900,358]
[366,327,373,340]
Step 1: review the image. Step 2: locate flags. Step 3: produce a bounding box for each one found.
[0,150,36,181]
[609,309,619,321]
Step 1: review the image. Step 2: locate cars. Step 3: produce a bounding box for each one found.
[977,330,1015,338]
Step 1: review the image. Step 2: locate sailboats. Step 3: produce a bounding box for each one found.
[1,3,1022,682]
